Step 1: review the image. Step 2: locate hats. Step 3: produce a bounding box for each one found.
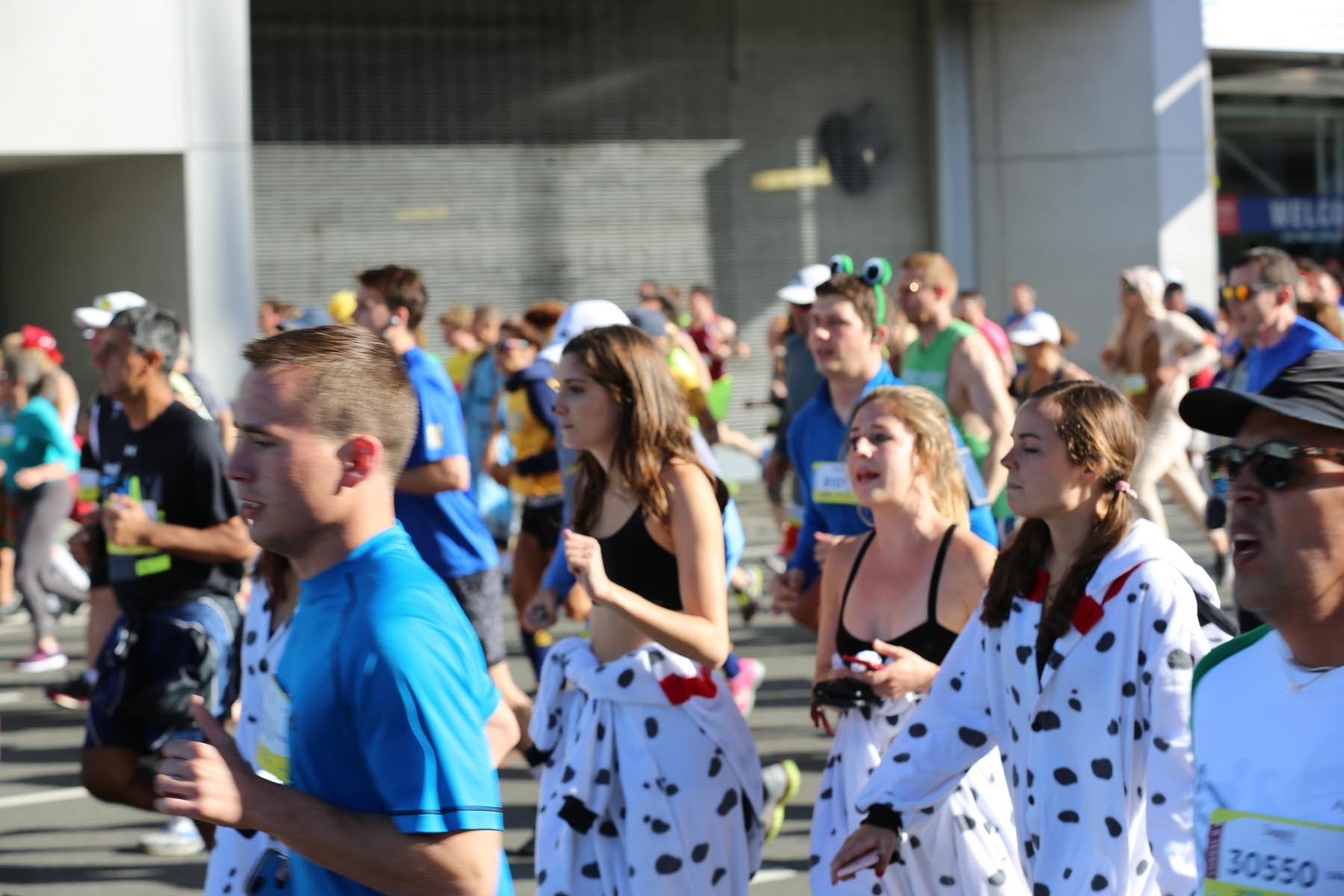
[775,263,832,305]
[19,324,63,366]
[1008,311,1062,346]
[536,299,631,365]
[72,290,147,327]
[277,306,334,333]
[1179,349,1344,438]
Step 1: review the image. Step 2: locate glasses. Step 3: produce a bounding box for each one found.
[894,283,931,299]
[1219,282,1284,304]
[1205,444,1344,493]
[495,338,535,354]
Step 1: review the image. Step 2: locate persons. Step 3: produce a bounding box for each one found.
[1,247,1343,841]
[1177,350,1344,896]
[810,386,1030,895]
[830,378,1238,896]
[150,325,514,896]
[526,326,765,895]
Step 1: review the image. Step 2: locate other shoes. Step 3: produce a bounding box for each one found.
[9,646,69,672]
[1214,545,1236,586]
[137,816,207,855]
[46,672,96,711]
[733,564,763,620]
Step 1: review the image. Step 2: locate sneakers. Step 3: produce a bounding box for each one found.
[725,656,767,716]
[759,760,800,845]
